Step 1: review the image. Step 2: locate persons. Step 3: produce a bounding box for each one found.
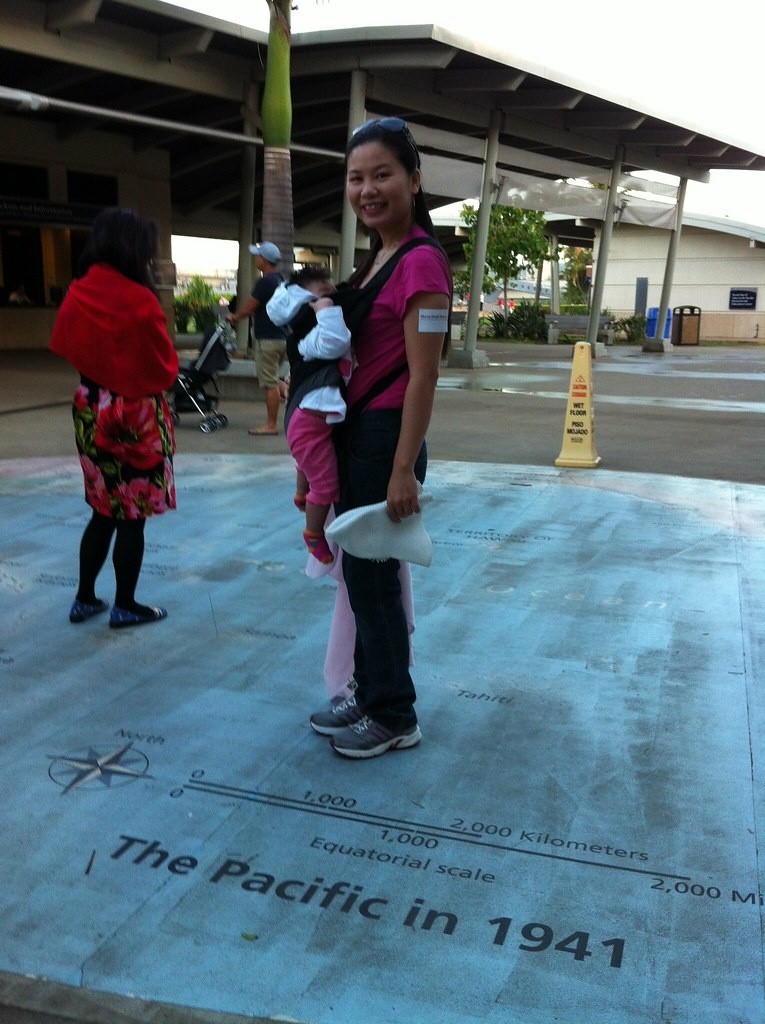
[224,240,288,436]
[48,212,178,630]
[266,116,453,756]
[463,291,514,314]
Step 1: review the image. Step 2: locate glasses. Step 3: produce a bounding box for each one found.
[352,118,417,151]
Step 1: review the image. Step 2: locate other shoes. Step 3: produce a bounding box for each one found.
[69,598,109,622]
[110,605,167,627]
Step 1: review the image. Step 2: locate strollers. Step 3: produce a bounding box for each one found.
[161,317,233,434]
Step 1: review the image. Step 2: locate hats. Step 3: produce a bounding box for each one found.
[249,241,280,265]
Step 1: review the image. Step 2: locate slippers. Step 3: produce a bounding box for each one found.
[248,427,278,435]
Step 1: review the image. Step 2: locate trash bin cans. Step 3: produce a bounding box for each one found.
[671,305,701,346]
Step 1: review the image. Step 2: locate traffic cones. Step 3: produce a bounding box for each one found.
[553,341,603,468]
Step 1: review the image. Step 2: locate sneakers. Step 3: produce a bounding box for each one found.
[310,694,365,736]
[328,715,422,758]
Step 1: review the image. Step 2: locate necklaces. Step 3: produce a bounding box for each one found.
[375,236,403,264]
